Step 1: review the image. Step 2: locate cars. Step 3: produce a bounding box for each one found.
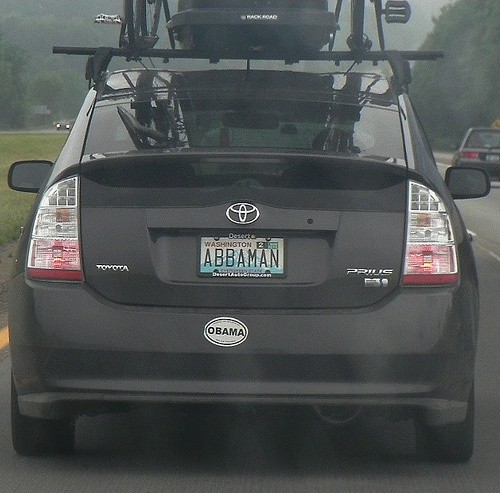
[7,67,492,462]
[56,121,70,132]
[454,118,500,187]
